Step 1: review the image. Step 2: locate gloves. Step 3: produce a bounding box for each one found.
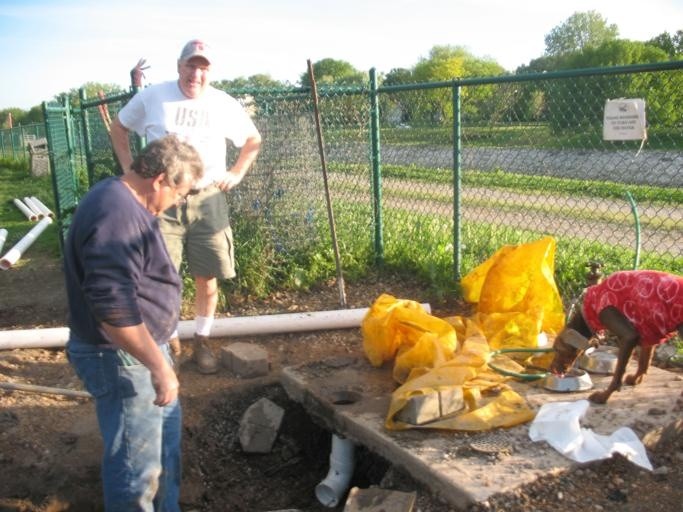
[130,59,150,86]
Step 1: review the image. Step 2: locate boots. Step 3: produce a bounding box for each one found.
[167,337,186,376]
[191,332,222,376]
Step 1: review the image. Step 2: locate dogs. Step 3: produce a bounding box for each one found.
[547,269,683,405]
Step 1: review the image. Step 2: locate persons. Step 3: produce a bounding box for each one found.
[109,39,262,375]
[61,133,205,512]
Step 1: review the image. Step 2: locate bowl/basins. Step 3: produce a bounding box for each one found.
[539,368,592,391]
[579,346,626,374]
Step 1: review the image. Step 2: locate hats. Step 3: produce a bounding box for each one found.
[182,38,215,66]
